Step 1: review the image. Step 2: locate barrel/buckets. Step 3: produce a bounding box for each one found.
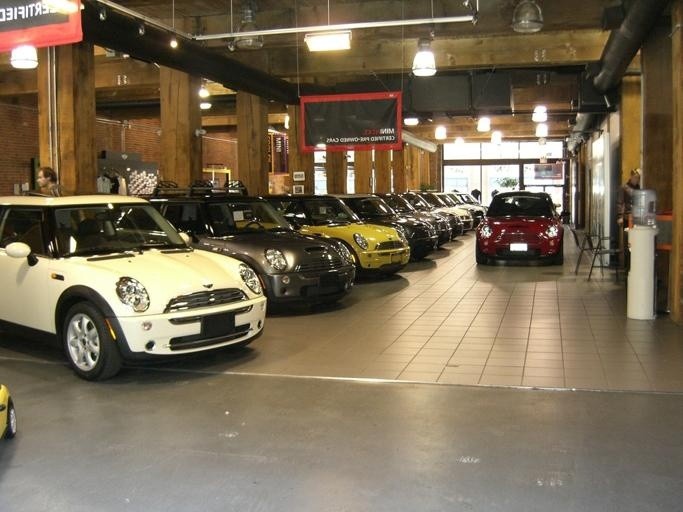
[632,188,658,226]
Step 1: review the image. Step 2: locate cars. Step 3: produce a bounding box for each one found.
[263,193,411,282]
[0,192,270,383]
[139,194,358,314]
[474,192,564,265]
[336,189,487,259]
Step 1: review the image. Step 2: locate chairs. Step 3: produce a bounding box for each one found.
[586,233,622,283]
[571,228,603,276]
[77,219,108,247]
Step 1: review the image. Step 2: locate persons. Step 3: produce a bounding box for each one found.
[492,189,499,200]
[36,166,69,198]
[471,189,481,202]
[616,169,641,271]
